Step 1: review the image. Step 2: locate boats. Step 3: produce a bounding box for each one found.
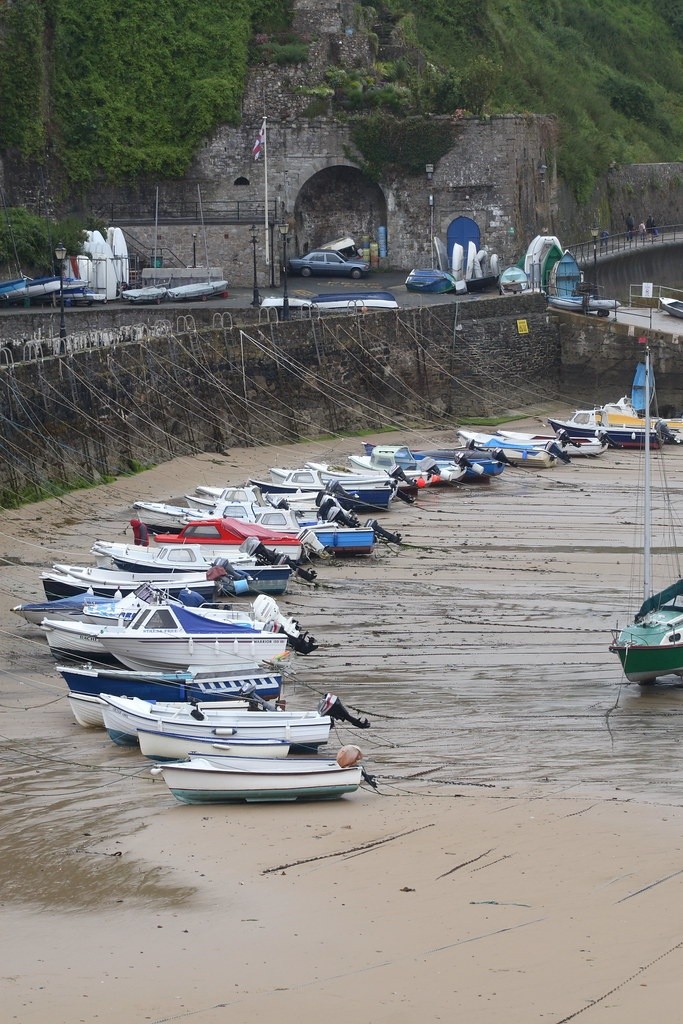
[154,744,377,806]
[55,650,297,728]
[403,234,502,296]
[135,726,292,762]
[9,495,403,673]
[62,225,129,300]
[99,692,372,755]
[195,358,683,503]
[498,225,683,318]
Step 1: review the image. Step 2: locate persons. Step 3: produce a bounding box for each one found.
[639,221,647,240]
[646,214,656,241]
[130,519,149,547]
[601,229,610,246]
[626,212,634,242]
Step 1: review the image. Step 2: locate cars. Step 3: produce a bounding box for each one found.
[287,248,371,279]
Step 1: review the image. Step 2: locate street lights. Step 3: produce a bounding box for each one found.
[248,224,266,307]
[53,240,68,355]
[277,217,291,322]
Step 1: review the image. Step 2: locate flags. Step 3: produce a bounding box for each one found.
[253,120,265,161]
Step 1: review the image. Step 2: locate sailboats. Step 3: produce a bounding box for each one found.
[122,183,228,305]
[0,164,108,308]
[607,344,683,685]
